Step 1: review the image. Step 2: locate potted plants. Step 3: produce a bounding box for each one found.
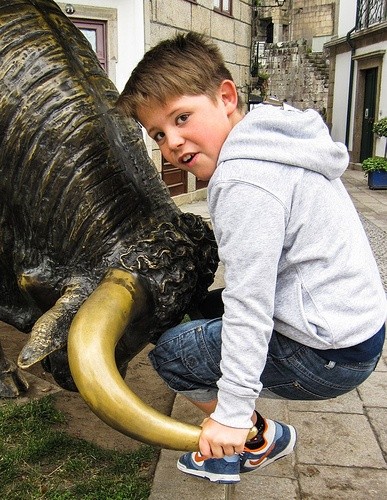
[361,156,387,190]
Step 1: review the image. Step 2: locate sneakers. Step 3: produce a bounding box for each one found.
[177,451,241,485]
[240,418,297,474]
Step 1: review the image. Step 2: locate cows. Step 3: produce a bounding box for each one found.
[0,0,258,454]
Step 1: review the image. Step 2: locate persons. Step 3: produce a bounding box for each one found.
[115,32,387,485]
